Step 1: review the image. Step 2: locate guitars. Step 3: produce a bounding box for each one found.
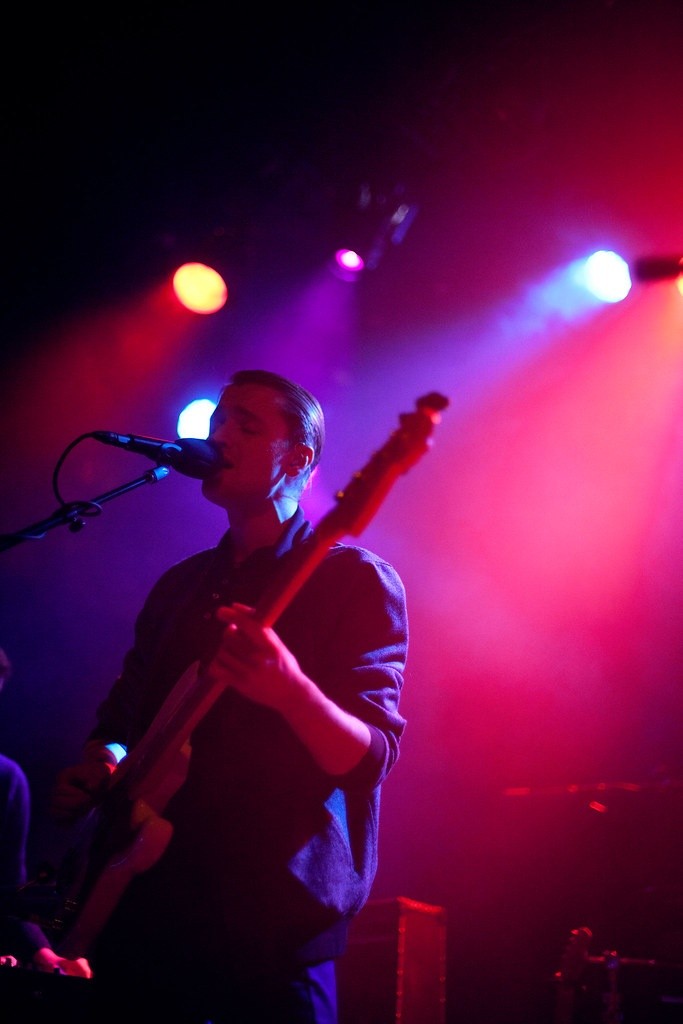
[29,388,451,960]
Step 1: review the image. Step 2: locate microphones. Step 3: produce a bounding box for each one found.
[90,430,222,480]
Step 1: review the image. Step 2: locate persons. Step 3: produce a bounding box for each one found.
[55,367,409,1024]
[0,647,92,980]
